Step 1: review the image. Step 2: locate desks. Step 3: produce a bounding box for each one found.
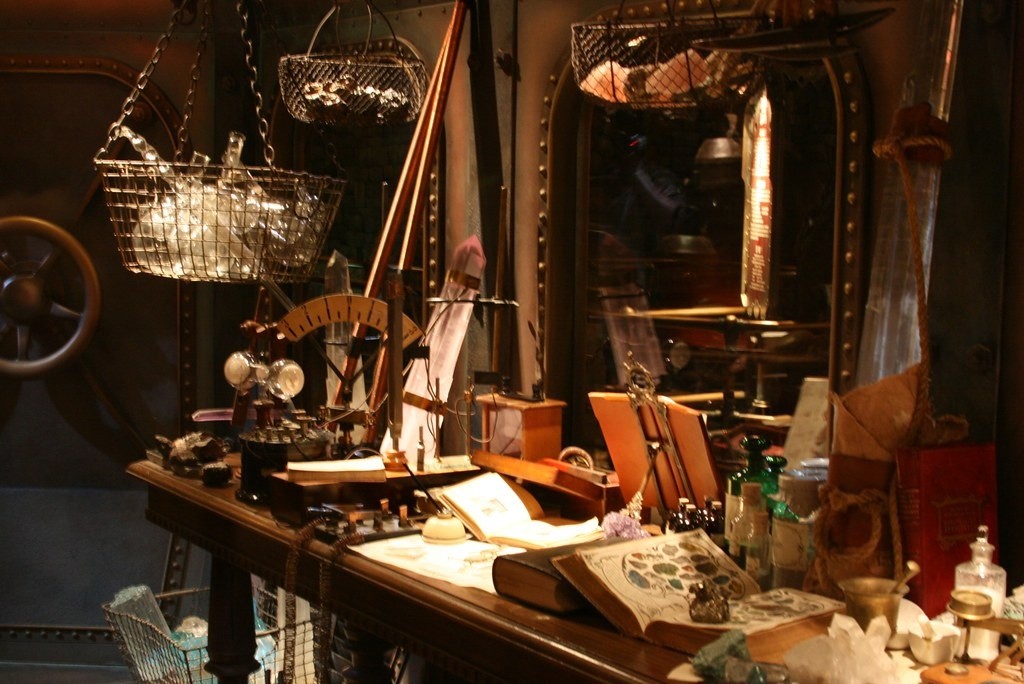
[122,454,1023,684]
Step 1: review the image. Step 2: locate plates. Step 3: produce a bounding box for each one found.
[422,534,473,544]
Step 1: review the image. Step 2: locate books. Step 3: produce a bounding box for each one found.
[438,472,604,550]
[897,445,1002,619]
[492,538,636,616]
[287,455,387,483]
[550,524,841,665]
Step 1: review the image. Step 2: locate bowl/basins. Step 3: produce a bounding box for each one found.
[422,513,466,539]
[909,622,960,665]
[888,598,929,649]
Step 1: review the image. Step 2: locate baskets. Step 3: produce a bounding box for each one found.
[568,10,772,113]
[103,584,326,684]
[94,157,344,283]
[277,50,430,131]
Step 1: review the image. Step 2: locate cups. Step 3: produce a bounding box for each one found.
[839,577,909,658]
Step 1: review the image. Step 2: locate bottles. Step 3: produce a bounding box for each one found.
[665,498,689,535]
[954,525,1006,662]
[701,495,715,520]
[725,436,778,569]
[675,503,700,533]
[729,483,762,571]
[745,512,771,591]
[705,501,724,551]
